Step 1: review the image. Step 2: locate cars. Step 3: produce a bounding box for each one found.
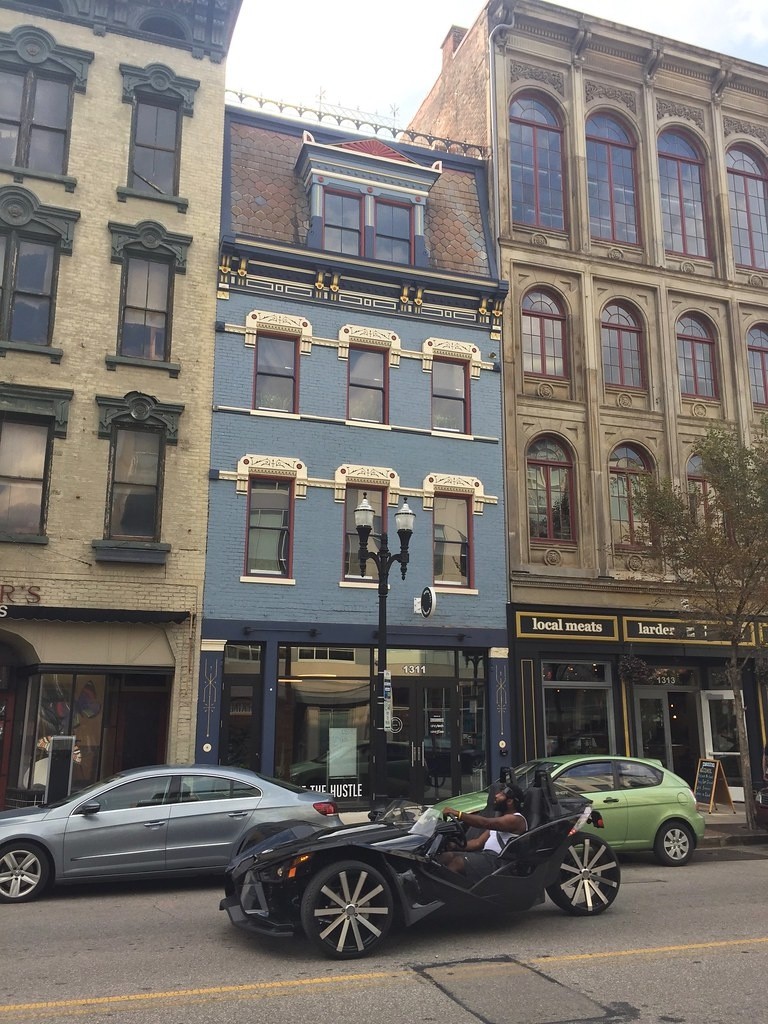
[0,763,347,905]
[403,735,488,781]
[279,739,432,802]
[644,724,740,783]
[432,753,707,868]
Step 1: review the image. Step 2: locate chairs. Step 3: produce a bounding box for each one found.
[182,782,191,802]
[524,787,542,830]
[464,782,505,842]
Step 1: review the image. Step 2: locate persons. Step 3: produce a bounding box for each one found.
[436,783,528,879]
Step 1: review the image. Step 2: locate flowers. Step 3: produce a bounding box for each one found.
[617,653,647,683]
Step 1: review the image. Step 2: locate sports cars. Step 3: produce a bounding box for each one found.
[212,762,620,961]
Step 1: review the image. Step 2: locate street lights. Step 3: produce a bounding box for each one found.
[352,489,418,800]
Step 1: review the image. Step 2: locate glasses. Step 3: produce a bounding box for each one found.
[501,783,515,800]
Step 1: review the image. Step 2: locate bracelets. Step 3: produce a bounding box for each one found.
[458,811,462,819]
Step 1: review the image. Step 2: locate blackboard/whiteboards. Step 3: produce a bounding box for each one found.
[44,735,75,804]
[329,728,358,778]
[694,759,733,806]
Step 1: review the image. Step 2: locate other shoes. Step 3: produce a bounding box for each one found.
[411,896,440,909]
